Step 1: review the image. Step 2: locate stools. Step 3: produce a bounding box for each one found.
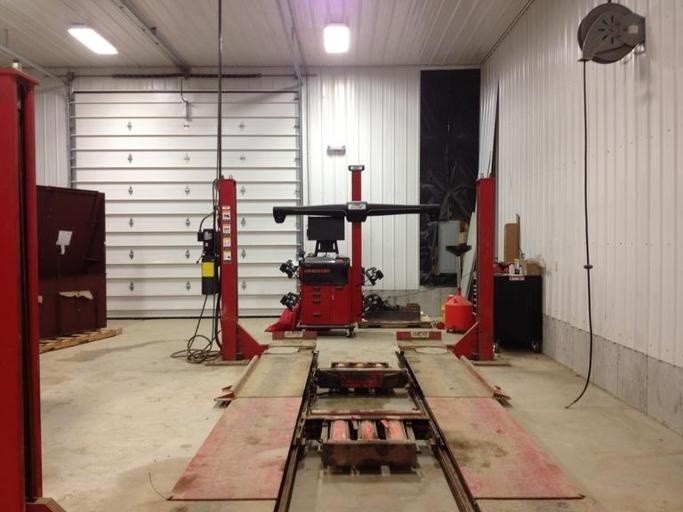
[445,244,472,298]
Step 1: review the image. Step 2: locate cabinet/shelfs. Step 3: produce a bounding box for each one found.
[36,185,106,340]
[472,272,542,352]
